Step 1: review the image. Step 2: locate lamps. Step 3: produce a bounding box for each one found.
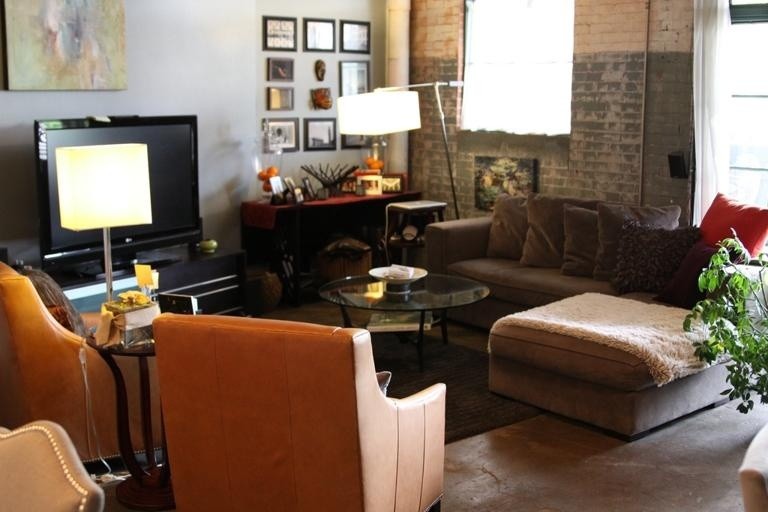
[335,80,464,221]
[56,145,148,332]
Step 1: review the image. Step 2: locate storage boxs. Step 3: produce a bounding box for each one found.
[159,273,241,316]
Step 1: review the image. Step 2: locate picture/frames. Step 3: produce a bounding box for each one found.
[303,119,337,150]
[267,60,296,82]
[268,84,294,107]
[264,118,299,152]
[342,20,372,53]
[267,169,407,205]
[340,63,369,101]
[342,119,372,148]
[303,17,337,50]
[262,16,297,48]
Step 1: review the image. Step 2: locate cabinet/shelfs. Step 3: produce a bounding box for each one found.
[29,248,255,329]
[383,196,449,265]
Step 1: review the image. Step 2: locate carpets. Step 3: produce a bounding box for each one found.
[350,340,547,447]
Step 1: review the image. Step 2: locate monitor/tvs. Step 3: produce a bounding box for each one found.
[34,114,202,279]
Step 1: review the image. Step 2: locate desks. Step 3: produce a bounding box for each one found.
[87,332,175,511]
[155,314,448,510]
[243,187,422,296]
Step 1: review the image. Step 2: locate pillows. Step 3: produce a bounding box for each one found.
[619,221,700,292]
[601,206,683,275]
[704,193,766,257]
[488,194,528,260]
[525,198,571,266]
[562,207,599,275]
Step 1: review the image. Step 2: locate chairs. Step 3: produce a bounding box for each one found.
[2,418,106,510]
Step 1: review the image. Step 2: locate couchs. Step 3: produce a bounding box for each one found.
[427,203,754,351]
[3,256,187,459]
[490,295,759,438]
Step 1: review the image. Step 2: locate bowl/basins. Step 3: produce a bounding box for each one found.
[367,264,428,294]
[376,294,427,309]
